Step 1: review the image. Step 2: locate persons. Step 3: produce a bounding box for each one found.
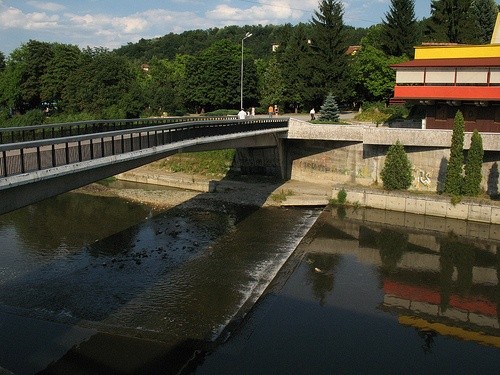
[274,105,279,118]
[238,108,247,120]
[268,105,273,119]
[309,107,315,121]
[251,106,256,117]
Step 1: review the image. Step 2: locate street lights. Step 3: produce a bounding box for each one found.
[240,32,253,110]
